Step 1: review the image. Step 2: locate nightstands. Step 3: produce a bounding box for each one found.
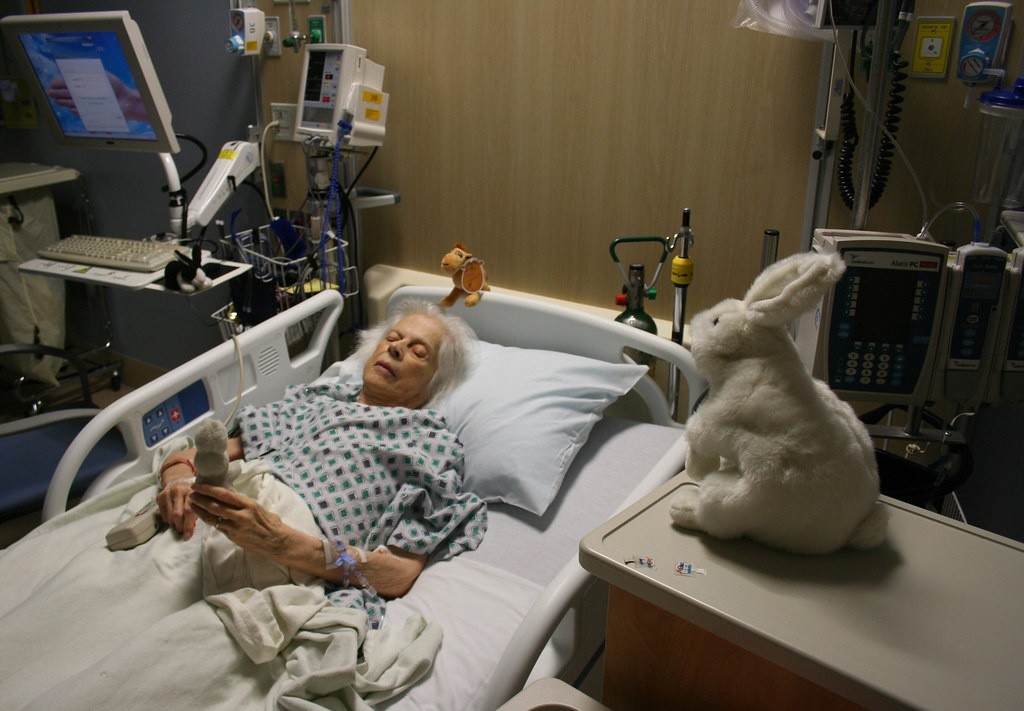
[580,463,1024,711]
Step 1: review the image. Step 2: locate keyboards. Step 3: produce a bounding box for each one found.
[37,234,191,271]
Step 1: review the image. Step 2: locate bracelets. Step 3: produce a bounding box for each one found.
[160,457,196,475]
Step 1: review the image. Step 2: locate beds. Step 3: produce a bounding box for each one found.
[0,285,708,711]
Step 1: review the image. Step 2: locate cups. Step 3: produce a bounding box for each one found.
[976,87,1024,208]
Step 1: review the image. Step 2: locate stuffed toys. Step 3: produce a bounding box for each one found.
[440,243,490,307]
[669,252,889,554]
[194,421,240,495]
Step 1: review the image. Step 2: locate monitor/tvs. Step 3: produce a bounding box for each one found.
[0,11,182,154]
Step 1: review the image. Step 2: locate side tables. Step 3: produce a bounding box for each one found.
[0,163,124,412]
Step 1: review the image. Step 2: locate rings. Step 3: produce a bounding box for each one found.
[215,518,223,529]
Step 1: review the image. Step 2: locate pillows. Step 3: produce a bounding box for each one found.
[319,333,650,516]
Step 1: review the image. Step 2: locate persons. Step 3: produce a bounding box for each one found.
[155,297,488,600]
